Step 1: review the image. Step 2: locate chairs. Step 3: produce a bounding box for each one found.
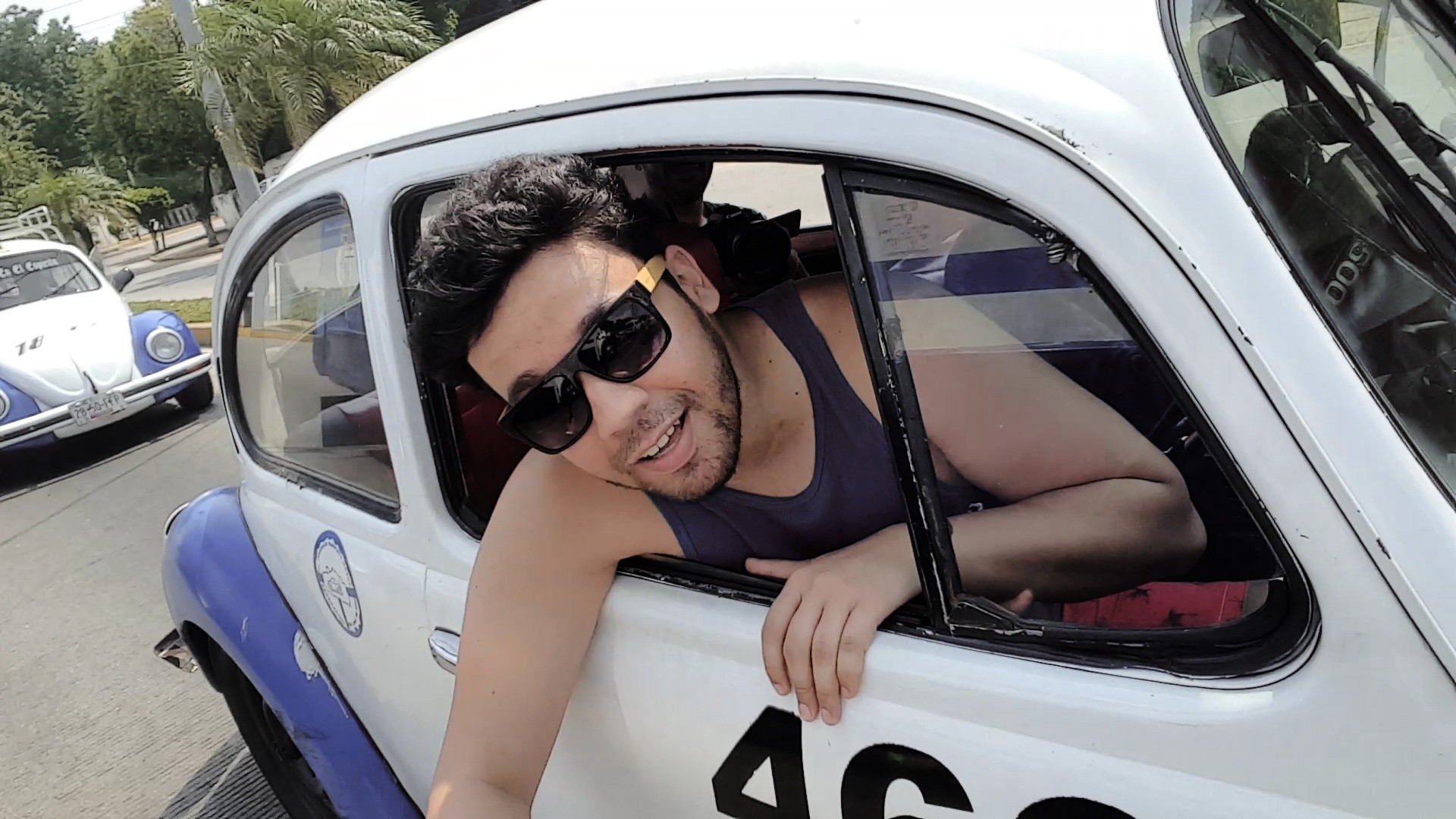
[280,383,529,524]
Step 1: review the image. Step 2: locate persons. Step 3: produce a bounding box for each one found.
[11,261,81,304]
[394,148,1209,819]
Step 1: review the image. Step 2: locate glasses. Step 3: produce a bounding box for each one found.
[497,256,672,454]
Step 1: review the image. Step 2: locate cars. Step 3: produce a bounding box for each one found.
[158,0,1453,819]
[0,239,215,454]
